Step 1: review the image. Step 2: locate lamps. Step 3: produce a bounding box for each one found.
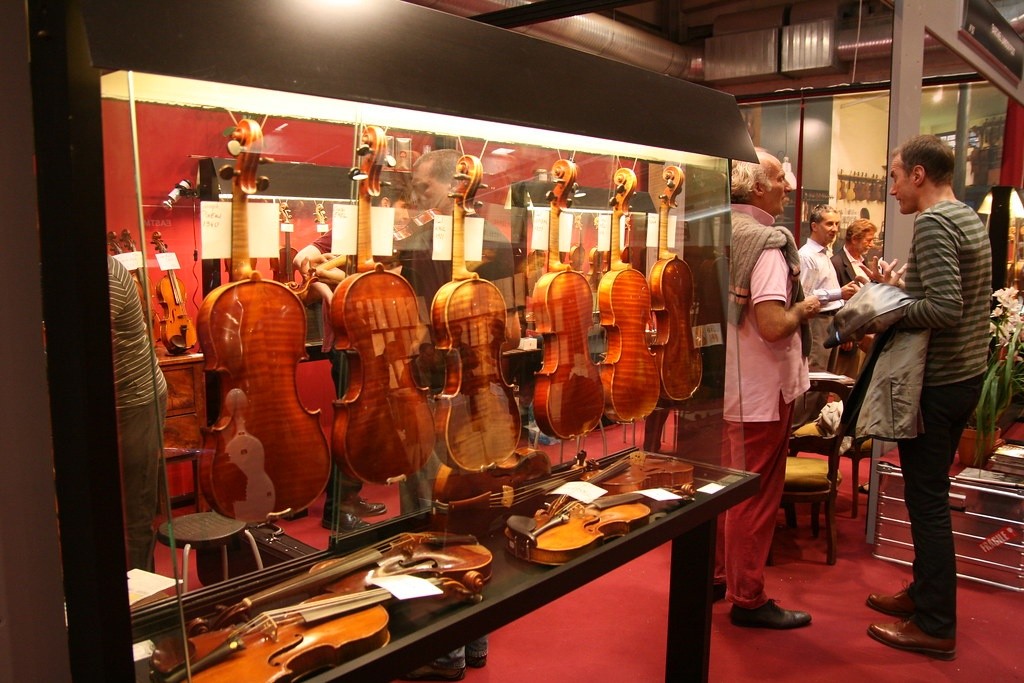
[782,104,803,191]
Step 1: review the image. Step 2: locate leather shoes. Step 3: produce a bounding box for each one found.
[346,498,387,517]
[712,583,727,602]
[867,616,956,660]
[730,598,811,629]
[867,579,915,616]
[322,511,371,533]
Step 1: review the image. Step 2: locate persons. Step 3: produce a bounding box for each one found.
[403,149,514,534]
[712,147,821,629]
[292,182,415,536]
[300,228,489,681]
[859,135,992,660]
[517,330,544,421]
[791,204,877,433]
[107,255,168,574]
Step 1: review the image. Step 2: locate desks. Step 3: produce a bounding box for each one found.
[154,345,210,513]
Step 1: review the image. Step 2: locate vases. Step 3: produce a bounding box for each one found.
[958,426,1001,466]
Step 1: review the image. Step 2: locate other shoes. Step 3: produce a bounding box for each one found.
[466,656,486,667]
[858,483,869,494]
[403,664,466,680]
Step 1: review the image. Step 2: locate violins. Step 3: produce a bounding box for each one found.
[525,251,545,296]
[501,449,696,565]
[621,216,635,264]
[650,164,703,402]
[568,215,611,292]
[151,230,197,354]
[303,203,337,312]
[149,570,484,683]
[107,228,147,322]
[431,447,600,537]
[835,170,888,204]
[198,119,331,521]
[534,158,605,439]
[600,167,661,421]
[186,531,493,638]
[431,153,521,473]
[268,202,300,284]
[224,257,257,280]
[321,125,437,485]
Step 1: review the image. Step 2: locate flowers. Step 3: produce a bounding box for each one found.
[973,287,1024,479]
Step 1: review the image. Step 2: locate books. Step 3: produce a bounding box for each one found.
[987,444,1024,475]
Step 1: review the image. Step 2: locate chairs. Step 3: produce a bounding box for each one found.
[788,422,873,519]
[765,379,852,566]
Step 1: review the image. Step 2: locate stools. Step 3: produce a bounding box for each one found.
[157,513,264,594]
